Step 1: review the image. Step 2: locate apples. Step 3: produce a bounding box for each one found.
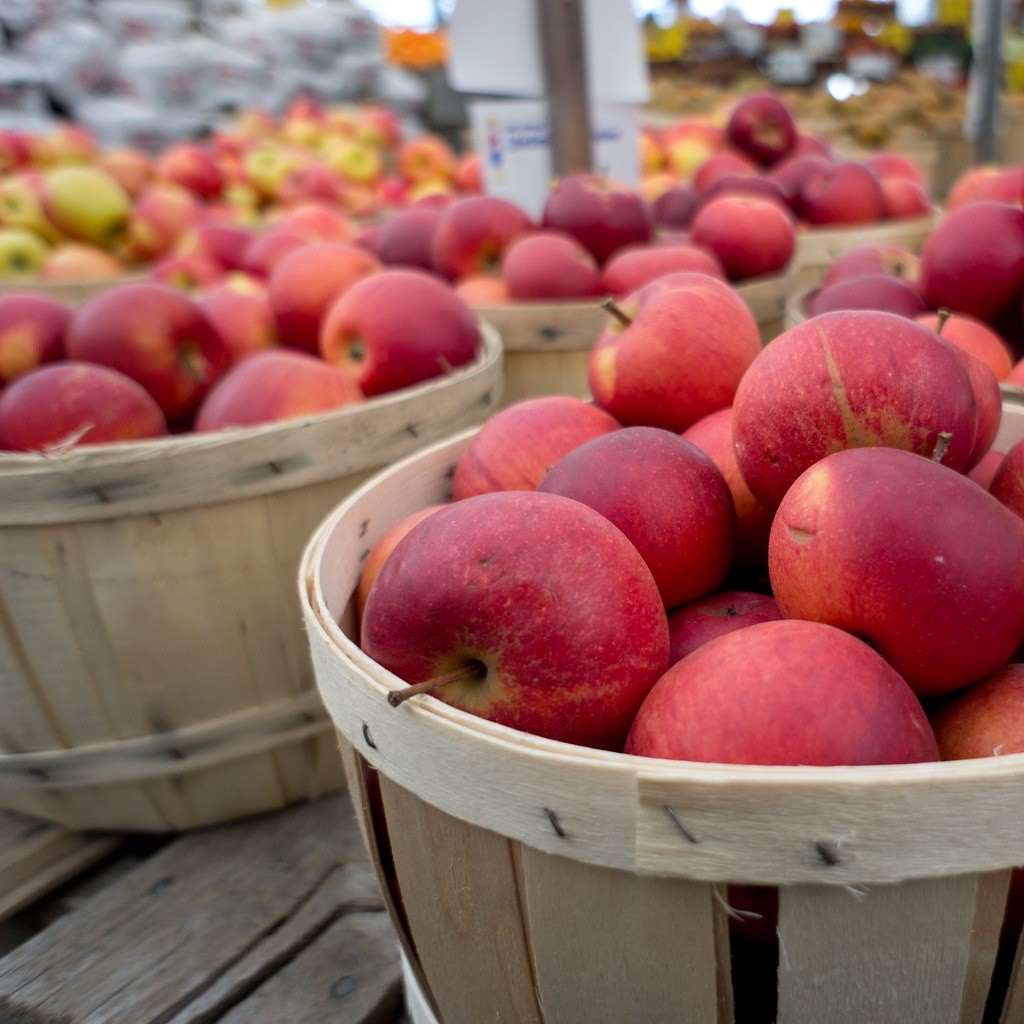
[0,92,1024,935]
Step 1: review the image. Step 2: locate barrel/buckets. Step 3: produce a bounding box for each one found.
[3,206,1016,1022]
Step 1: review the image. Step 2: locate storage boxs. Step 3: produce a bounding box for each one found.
[0,97,1024,1023]
